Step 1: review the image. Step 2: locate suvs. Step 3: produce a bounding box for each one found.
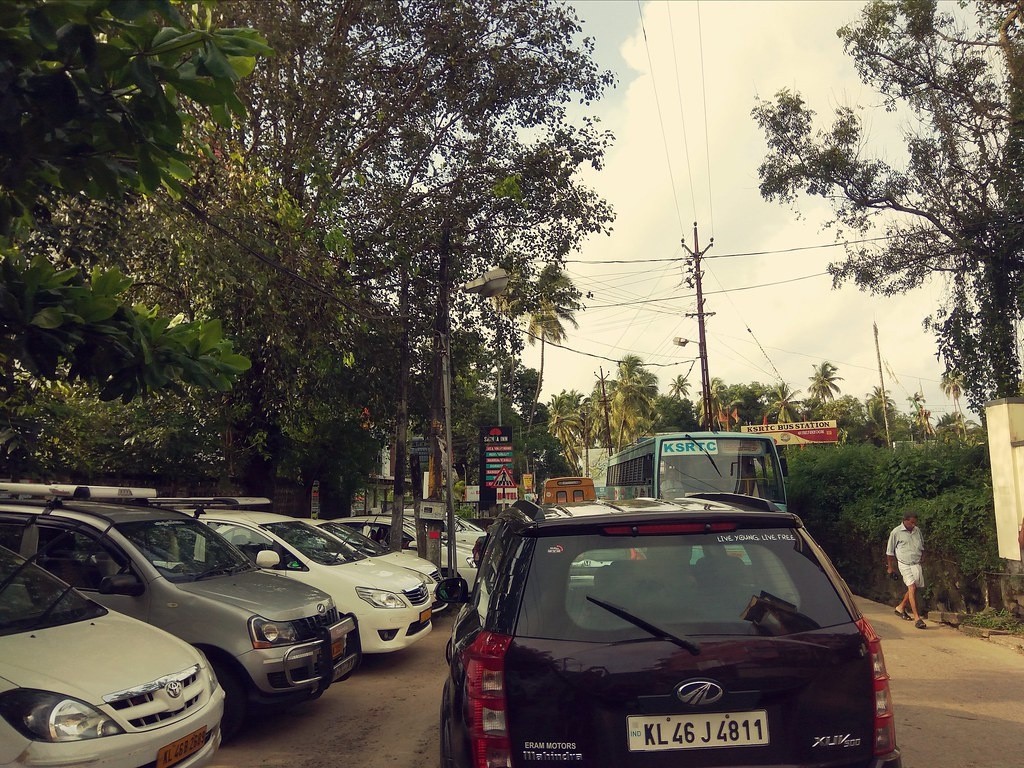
[1,482,361,722]
[439,492,904,768]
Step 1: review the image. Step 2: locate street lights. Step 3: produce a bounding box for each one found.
[672,336,720,430]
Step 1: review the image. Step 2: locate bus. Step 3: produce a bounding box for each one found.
[600,429,790,568]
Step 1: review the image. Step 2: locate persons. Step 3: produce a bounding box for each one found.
[886,509,929,629]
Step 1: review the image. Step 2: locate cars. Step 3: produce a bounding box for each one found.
[156,499,434,654]
[214,518,455,615]
[0,543,228,768]
[330,507,491,602]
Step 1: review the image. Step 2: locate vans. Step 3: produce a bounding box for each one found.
[542,477,598,508]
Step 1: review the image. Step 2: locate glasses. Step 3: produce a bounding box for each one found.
[474,560,479,565]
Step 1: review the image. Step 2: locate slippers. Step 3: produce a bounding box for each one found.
[915,619,926,628]
[895,610,912,621]
